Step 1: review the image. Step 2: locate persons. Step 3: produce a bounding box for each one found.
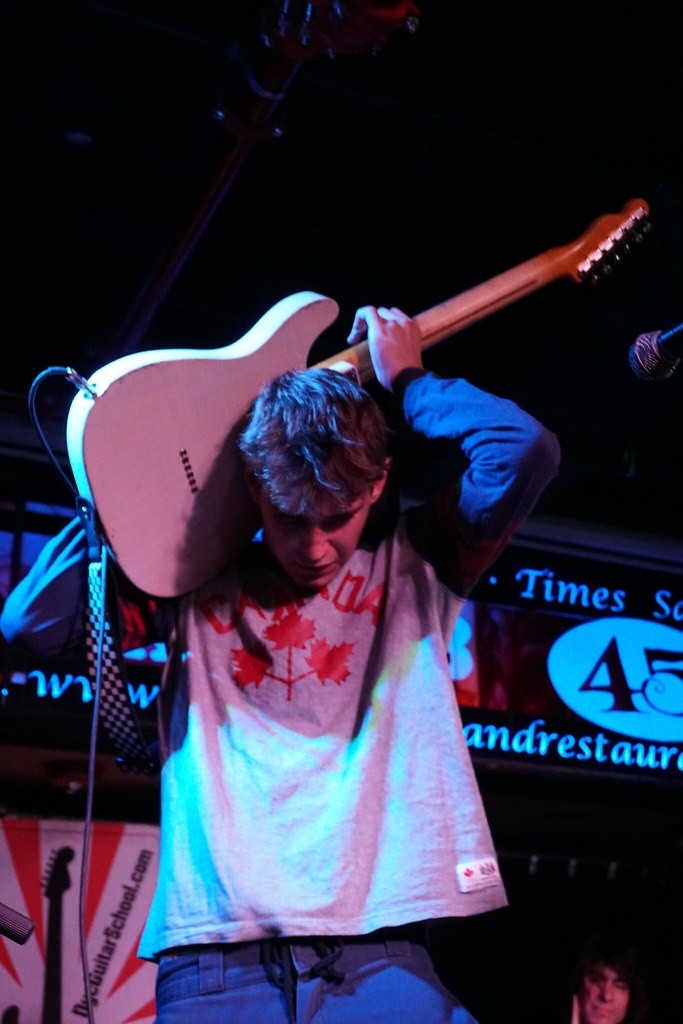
[571,947,634,1024]
[0,302,560,1023]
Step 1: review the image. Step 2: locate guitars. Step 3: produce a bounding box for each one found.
[62,195,655,607]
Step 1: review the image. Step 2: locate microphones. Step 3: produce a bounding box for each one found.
[630,324,683,382]
